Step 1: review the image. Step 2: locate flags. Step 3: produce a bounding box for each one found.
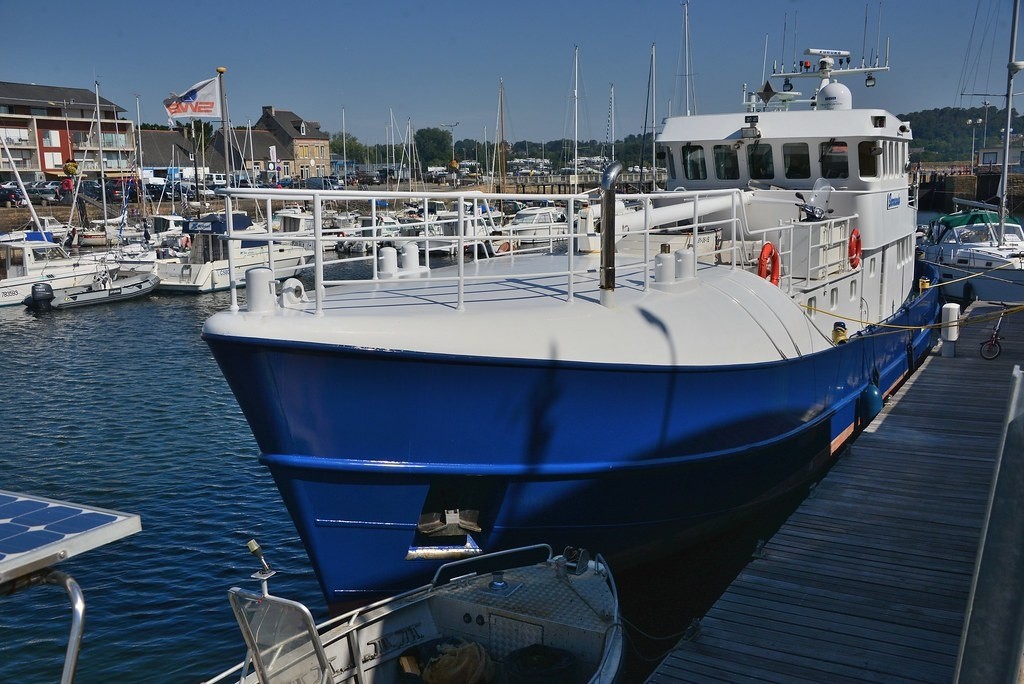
[163,77,223,118]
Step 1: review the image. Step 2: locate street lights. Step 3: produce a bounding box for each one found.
[981,99,991,148]
[440,122,460,167]
[965,118,982,176]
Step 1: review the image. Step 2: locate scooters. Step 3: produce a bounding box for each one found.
[794,176,834,223]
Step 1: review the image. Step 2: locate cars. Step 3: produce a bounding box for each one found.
[1,181,18,190]
[0,188,28,206]
[80,179,102,191]
[305,176,343,190]
[144,183,182,202]
[183,182,216,201]
[25,188,63,206]
[37,181,62,190]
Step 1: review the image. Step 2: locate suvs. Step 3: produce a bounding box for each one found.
[105,178,133,202]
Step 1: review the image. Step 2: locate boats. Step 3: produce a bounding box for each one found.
[10,0,1024,610]
[192,544,625,684]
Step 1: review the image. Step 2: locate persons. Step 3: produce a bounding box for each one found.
[54,187,60,202]
[15,185,22,208]
[541,170,544,176]
[516,171,520,176]
[529,170,534,176]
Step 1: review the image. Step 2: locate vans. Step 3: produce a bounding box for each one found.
[203,173,236,187]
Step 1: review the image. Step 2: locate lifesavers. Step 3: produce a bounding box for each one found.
[182,237,191,249]
[61,178,72,191]
[848,228,862,269]
[758,242,780,286]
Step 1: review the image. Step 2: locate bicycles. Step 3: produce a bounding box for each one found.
[980,301,1008,359]
